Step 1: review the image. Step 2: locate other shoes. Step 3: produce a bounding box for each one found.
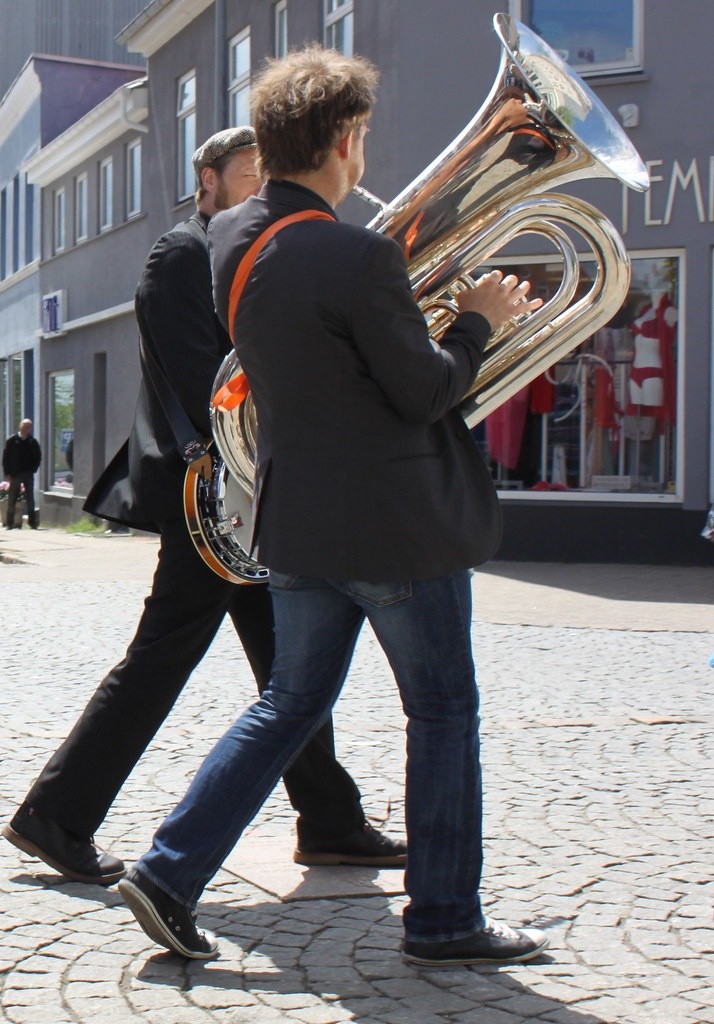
[29,523,37,529]
[6,522,15,530]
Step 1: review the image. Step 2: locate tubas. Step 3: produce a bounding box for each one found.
[210,12,651,501]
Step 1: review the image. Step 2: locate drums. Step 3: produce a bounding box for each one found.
[183,438,271,586]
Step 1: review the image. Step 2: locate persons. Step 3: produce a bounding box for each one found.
[627,280,679,406]
[115,44,549,963]
[1,418,42,531]
[0,124,409,884]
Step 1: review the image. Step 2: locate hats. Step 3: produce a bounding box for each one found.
[191,125,258,173]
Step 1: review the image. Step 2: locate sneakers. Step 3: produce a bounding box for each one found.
[118,867,220,959]
[402,917,550,966]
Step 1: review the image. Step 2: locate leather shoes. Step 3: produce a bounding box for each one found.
[293,818,408,866]
[2,801,127,884]
